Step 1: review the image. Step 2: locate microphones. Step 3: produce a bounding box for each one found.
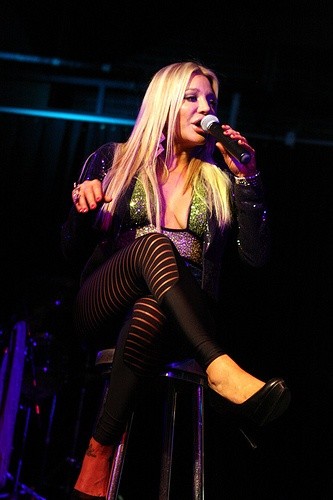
[200,114,251,164]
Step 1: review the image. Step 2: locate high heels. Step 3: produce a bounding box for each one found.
[205,376,286,454]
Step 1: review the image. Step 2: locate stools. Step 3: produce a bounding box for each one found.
[93,347,209,498]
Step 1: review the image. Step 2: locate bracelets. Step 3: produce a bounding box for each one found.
[233,170,264,187]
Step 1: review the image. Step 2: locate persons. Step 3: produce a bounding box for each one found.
[59,59,289,500]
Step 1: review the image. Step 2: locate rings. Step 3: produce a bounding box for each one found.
[73,181,81,202]
